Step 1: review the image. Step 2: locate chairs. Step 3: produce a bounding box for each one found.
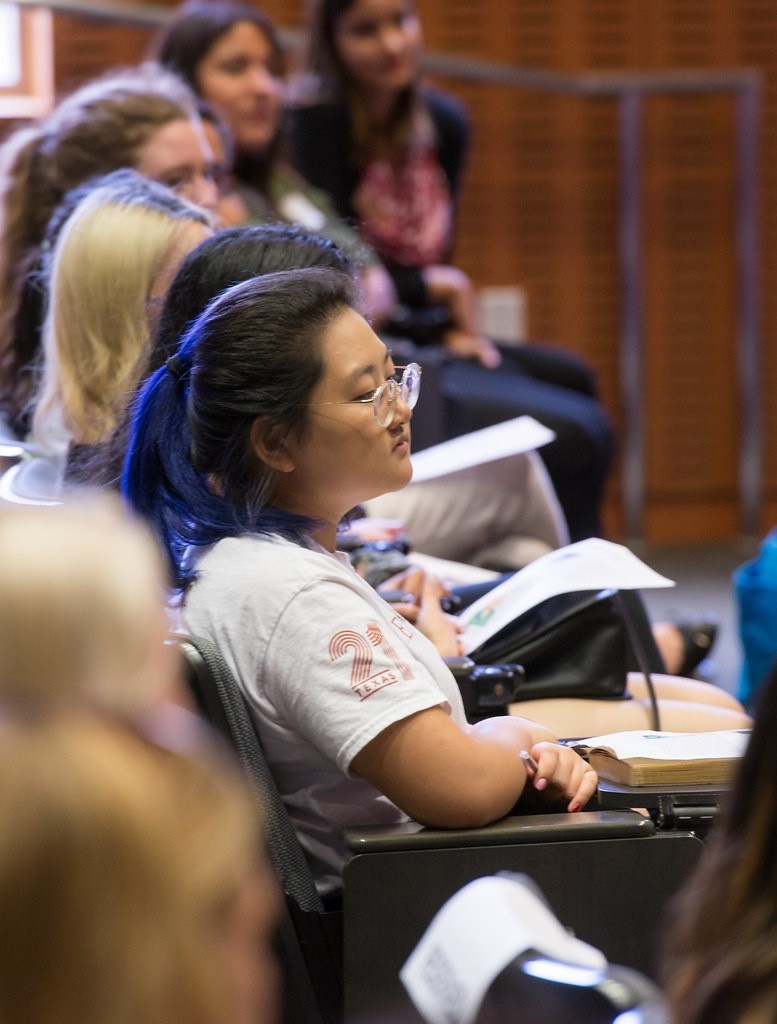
[176,625,703,1024]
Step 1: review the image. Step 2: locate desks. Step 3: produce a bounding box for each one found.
[558,736,739,807]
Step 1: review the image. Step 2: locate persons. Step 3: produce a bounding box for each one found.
[0,0,777,1024]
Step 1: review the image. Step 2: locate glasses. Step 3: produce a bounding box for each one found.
[292,362,423,427]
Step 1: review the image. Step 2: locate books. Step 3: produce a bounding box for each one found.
[568,724,741,787]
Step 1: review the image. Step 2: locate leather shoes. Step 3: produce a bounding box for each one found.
[664,621,721,677]
